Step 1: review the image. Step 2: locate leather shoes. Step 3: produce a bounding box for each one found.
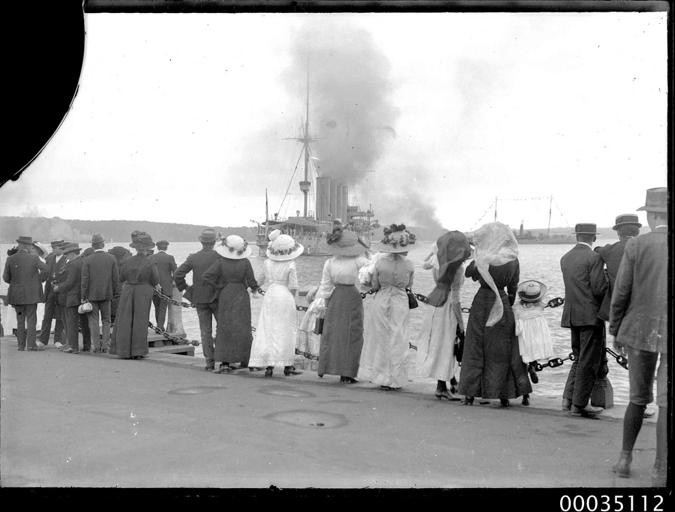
[284,369,303,376]
[436,366,603,417]
[17,341,107,354]
[265,370,272,376]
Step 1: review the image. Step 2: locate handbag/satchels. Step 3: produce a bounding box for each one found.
[453,323,465,363]
[407,293,418,309]
[313,312,323,334]
[78,300,93,314]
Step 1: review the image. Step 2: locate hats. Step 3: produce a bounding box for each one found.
[16,230,168,255]
[197,219,414,262]
[517,280,546,303]
[572,188,667,235]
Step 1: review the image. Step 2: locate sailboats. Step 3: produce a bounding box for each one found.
[472,192,576,245]
[255,64,387,256]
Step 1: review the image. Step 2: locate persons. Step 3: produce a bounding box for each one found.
[173,228,304,378]
[609,187,667,487]
[560,222,608,418]
[595,215,656,418]
[3,230,178,360]
[300,222,555,408]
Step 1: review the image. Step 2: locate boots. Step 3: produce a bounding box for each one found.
[612,451,632,478]
